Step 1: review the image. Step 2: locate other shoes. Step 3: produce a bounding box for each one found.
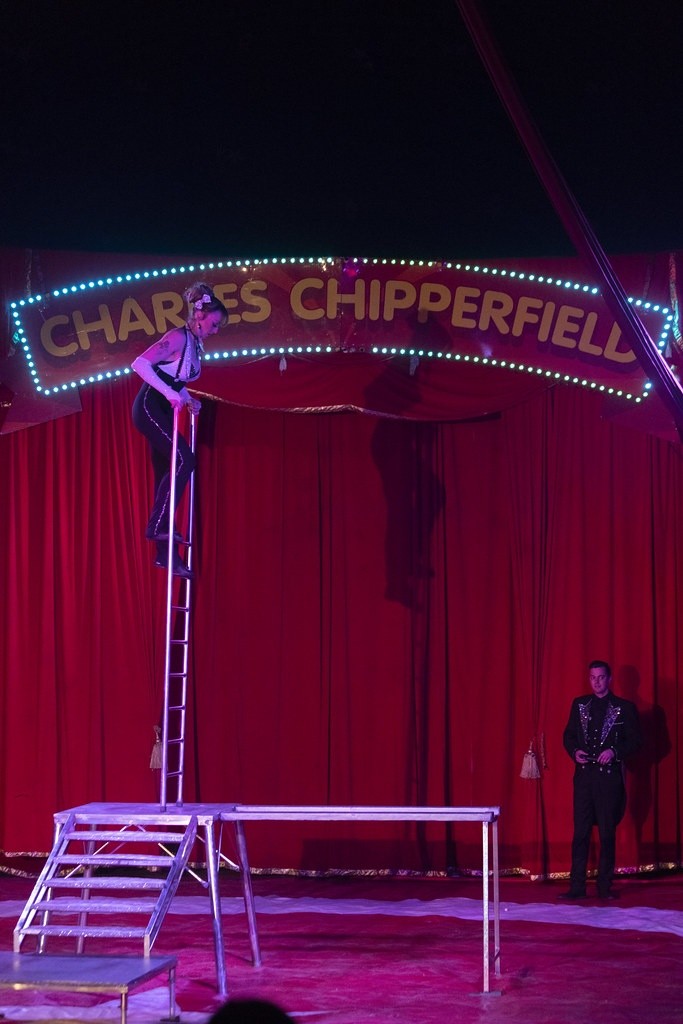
[598,888,618,900]
[154,555,192,578]
[558,889,588,899]
[147,530,182,543]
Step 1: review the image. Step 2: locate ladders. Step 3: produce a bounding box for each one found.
[160,393,196,813]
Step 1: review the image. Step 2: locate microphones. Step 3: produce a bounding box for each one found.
[581,754,613,764]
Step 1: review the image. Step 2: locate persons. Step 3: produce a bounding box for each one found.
[556,660,643,900]
[132,283,229,579]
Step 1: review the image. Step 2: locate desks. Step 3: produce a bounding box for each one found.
[0,950,179,1024]
